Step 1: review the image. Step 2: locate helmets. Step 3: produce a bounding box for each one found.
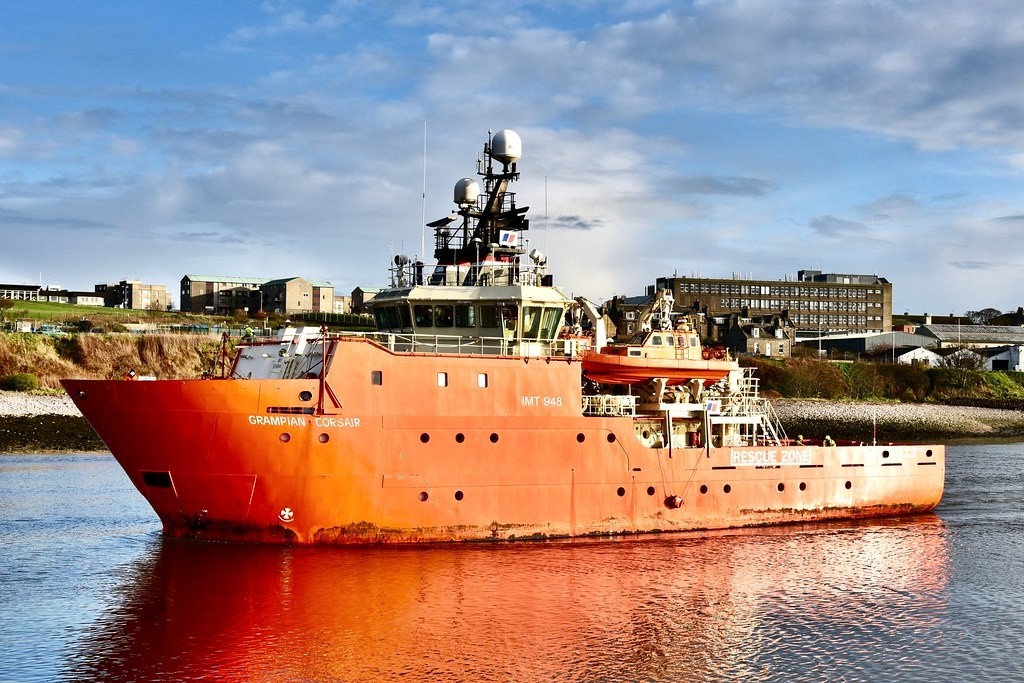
[243,325,248,328]
[285,320,291,325]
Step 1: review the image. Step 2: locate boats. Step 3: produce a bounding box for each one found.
[576,318,738,390]
[58,121,947,550]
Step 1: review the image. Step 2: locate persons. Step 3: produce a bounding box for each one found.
[795,435,805,445]
[244,325,258,343]
[822,435,836,447]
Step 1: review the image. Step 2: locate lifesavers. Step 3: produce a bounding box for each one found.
[701,347,713,360]
[713,345,725,359]
[732,391,743,405]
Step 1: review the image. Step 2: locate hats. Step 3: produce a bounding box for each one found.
[825,435,830,441]
[797,435,804,440]
[130,370,135,373]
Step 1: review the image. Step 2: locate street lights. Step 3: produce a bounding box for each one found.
[259,290,263,313]
[117,286,124,301]
